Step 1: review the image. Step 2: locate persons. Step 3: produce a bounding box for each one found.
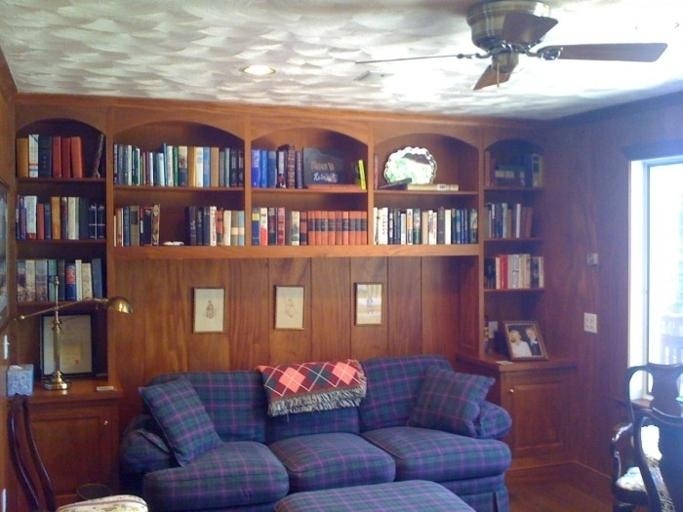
[525,325,539,356]
[509,330,532,356]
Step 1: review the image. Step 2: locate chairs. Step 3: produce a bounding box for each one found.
[605,359,682,512]
[5,386,150,511]
[632,405,682,512]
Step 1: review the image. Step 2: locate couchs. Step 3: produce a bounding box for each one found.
[120,352,517,512]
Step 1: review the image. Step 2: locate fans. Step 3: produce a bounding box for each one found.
[351,1,672,92]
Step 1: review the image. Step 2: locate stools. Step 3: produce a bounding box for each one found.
[274,476,480,512]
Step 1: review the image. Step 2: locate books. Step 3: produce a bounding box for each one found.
[113,140,367,247]
[13,133,103,302]
[374,207,479,246]
[483,148,547,288]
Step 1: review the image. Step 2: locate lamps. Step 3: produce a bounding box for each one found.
[13,274,133,393]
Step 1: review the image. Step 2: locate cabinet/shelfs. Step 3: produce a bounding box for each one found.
[453,124,550,357]
[11,246,109,386]
[1,385,124,512]
[452,355,577,477]
[14,96,482,260]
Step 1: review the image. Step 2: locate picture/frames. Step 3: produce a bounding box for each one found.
[37,313,101,379]
[273,283,307,331]
[352,280,385,326]
[190,286,226,334]
[502,319,550,361]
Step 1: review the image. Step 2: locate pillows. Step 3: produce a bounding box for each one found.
[404,363,499,439]
[137,372,226,467]
